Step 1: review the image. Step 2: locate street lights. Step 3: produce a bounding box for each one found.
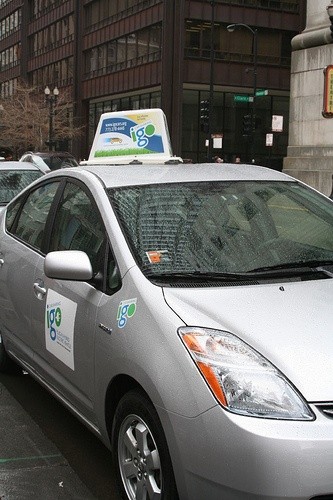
[44,85,60,153]
[227,24,258,135]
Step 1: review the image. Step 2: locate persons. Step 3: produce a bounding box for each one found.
[215,154,221,163]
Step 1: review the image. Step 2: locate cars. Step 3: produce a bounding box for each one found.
[0,151,80,210]
[0,109,333,500]
